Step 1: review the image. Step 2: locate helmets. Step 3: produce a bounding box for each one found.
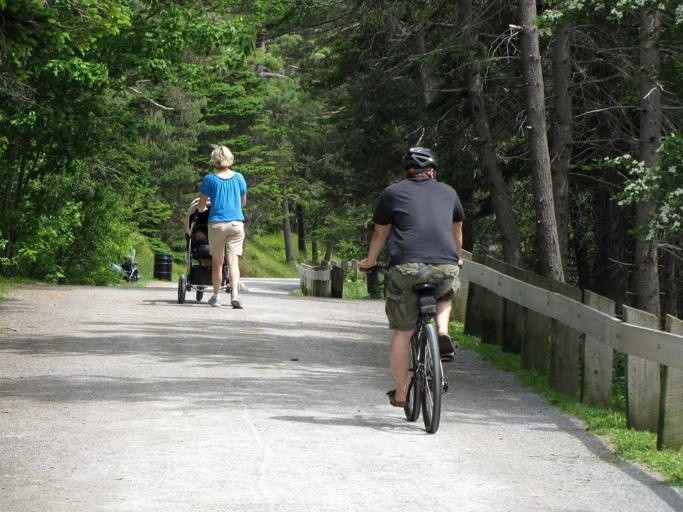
[403,147,440,171]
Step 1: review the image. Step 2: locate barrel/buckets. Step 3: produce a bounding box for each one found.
[154,253,172,281]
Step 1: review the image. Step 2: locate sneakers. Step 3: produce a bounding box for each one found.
[207,296,223,307]
[231,295,244,310]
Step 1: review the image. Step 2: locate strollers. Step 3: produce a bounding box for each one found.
[176,194,241,304]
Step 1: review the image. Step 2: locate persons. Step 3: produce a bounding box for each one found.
[357,146,466,407]
[198,145,246,309]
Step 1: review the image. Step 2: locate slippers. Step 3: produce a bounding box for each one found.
[437,333,457,354]
[387,389,409,407]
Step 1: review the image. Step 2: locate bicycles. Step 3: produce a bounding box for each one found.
[356,258,465,436]
